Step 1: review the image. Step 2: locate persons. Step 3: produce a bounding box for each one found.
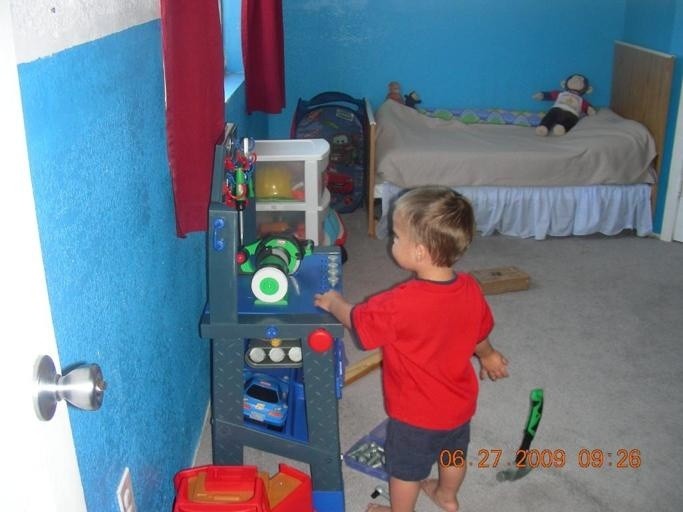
[314,184,509,512]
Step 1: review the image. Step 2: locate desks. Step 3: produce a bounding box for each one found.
[200,247,344,512]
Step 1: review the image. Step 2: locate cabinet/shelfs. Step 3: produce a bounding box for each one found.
[250,139,332,245]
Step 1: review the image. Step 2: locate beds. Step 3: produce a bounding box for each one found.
[363,41,676,240]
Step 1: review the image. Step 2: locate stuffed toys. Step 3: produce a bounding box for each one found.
[404,92,421,108]
[384,82,404,105]
[532,74,596,137]
